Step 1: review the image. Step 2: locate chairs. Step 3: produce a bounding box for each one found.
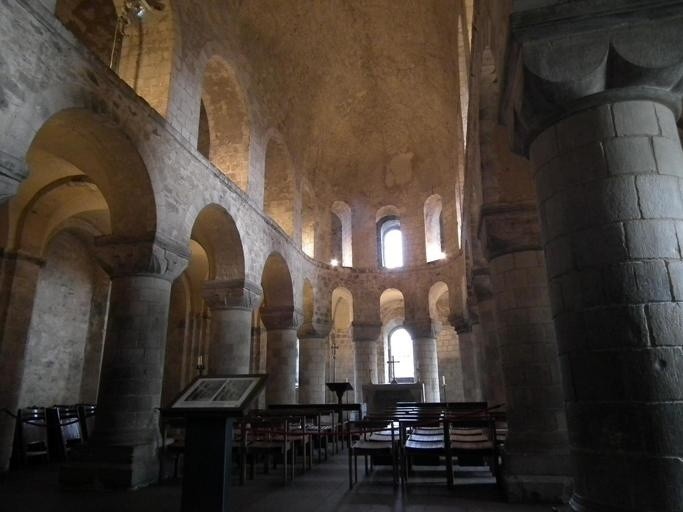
[158,403,513,491]
[17,402,99,473]
